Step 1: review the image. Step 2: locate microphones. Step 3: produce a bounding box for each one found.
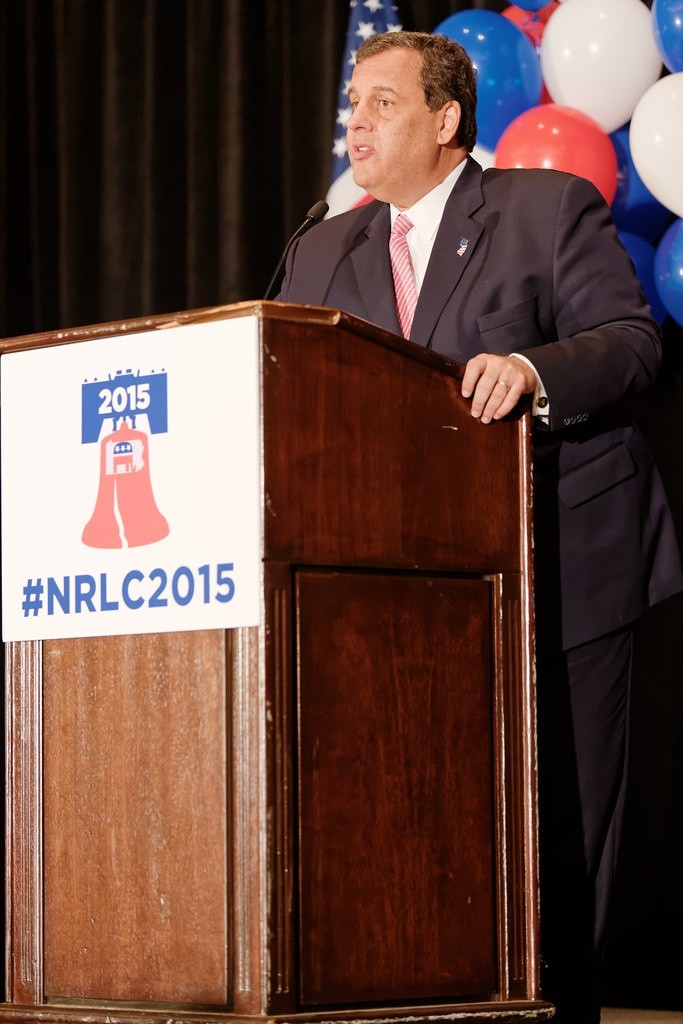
[264,200,329,299]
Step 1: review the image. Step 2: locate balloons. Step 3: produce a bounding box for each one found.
[432,10,542,153]
[651,0,683,73]
[493,105,618,207]
[540,0,663,135]
[500,0,560,47]
[654,219,683,324]
[617,229,666,324]
[629,72,683,217]
[606,130,670,237]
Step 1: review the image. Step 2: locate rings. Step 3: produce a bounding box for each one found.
[497,380,511,390]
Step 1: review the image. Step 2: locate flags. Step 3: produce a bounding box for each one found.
[323,0,404,221]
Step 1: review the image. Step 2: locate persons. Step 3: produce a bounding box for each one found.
[275,33,683,1023]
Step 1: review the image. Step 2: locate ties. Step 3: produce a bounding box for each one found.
[389,214,418,341]
